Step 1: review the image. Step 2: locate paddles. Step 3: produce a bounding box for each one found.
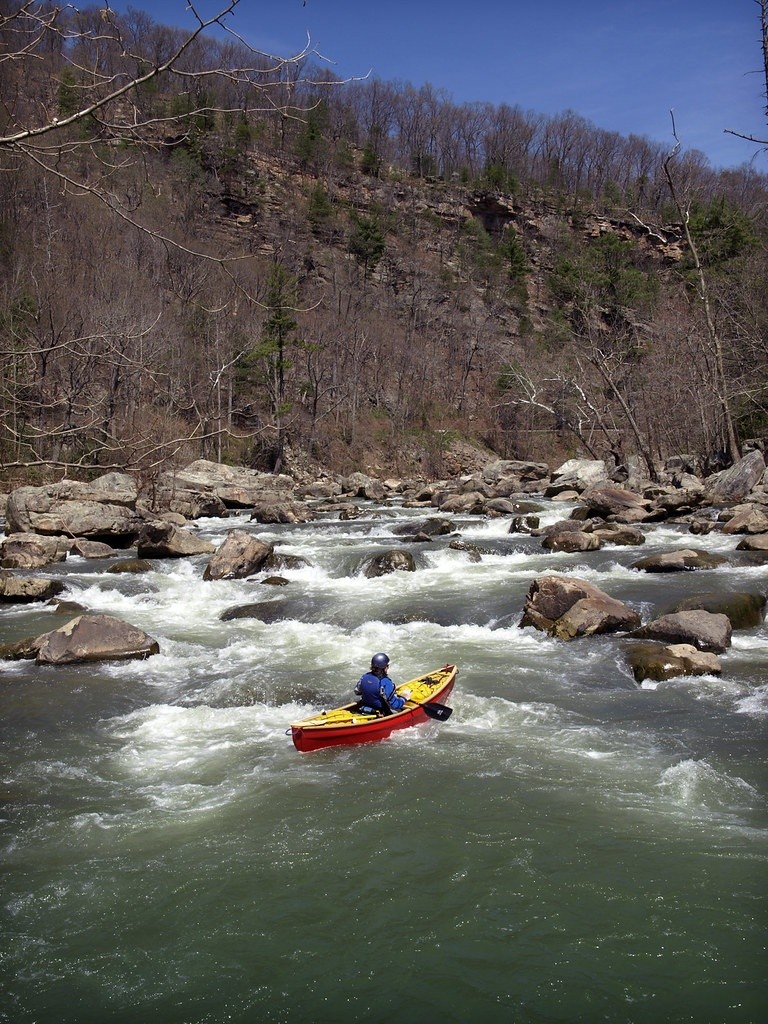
[395,695,453,722]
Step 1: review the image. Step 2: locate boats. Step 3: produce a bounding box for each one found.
[285,663,460,753]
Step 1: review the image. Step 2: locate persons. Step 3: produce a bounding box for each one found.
[354,653,412,718]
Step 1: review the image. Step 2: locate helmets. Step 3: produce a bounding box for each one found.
[372,653,390,668]
